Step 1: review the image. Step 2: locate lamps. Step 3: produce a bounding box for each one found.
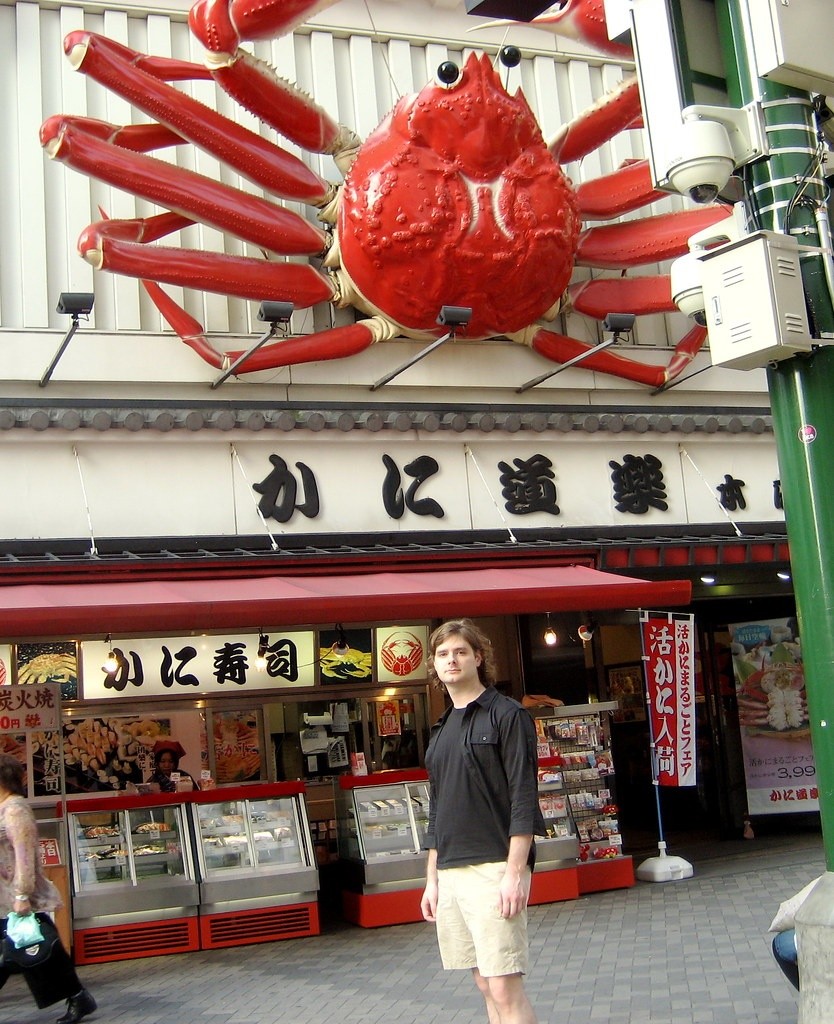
[209,299,293,391]
[577,625,595,641]
[517,313,635,395]
[38,295,95,391]
[370,306,472,393]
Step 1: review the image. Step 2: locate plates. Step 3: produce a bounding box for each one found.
[745,726,810,738]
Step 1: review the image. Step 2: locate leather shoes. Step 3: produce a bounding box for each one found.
[56,988,98,1024]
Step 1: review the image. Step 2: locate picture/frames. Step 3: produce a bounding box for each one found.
[206,704,267,784]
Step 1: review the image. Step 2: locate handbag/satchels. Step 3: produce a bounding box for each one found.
[7,910,45,949]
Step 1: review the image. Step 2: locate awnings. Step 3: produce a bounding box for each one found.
[0,559,697,640]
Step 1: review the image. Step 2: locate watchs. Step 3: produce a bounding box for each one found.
[16,895,30,901]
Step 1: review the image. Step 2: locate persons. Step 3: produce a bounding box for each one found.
[145,740,200,793]
[420,619,548,1024]
[0,755,97,1024]
[702,734,728,839]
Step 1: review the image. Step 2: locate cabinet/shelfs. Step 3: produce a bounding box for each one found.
[526,757,584,907]
[59,781,320,966]
[339,769,431,927]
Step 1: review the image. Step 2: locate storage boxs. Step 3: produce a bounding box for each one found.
[360,796,430,816]
[198,779,217,790]
[176,780,193,792]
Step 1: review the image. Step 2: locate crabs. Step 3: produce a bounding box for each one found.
[737,653,809,732]
[320,646,373,678]
[18,653,77,684]
[38,0,737,389]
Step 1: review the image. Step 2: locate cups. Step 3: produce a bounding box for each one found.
[177,782,193,792]
[135,782,151,793]
[760,647,770,660]
[771,626,792,642]
[731,642,745,675]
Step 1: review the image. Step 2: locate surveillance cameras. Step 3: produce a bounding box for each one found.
[666,120,737,205]
[670,250,708,327]
